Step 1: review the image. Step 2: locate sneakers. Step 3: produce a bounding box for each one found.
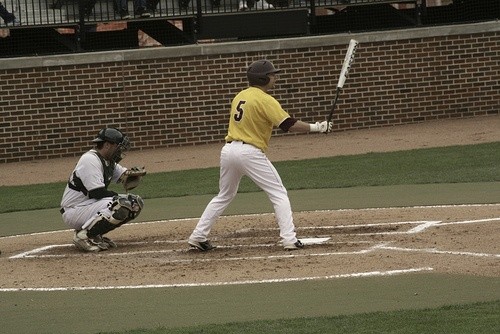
[188,238,216,252]
[73,230,111,253]
[284,240,304,249]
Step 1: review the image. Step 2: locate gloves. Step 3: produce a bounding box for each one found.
[310,120,333,133]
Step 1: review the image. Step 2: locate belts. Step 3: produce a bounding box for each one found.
[59,207,65,214]
[227,141,249,144]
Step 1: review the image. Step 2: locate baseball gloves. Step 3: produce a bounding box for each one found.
[120,167,146,190]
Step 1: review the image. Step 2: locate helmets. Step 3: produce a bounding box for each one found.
[92,127,125,145]
[247,60,282,86]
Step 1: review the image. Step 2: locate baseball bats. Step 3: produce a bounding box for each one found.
[325,39,359,135]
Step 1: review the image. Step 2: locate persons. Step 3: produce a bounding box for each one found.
[238,0,274,10]
[60,126,146,253]
[188,59,334,249]
[116,0,150,20]
